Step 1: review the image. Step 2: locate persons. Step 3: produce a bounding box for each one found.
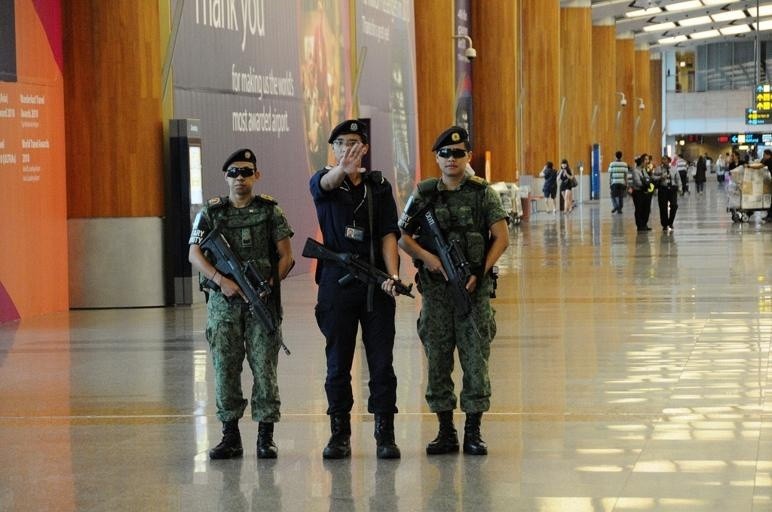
[537,149,772,232]
[187,147,295,461]
[541,223,682,304]
[396,125,511,456]
[309,120,401,460]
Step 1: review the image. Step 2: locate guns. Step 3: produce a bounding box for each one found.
[410,203,473,316]
[301,237,414,313]
[198,226,291,355]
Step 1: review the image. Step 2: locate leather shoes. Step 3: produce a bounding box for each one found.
[611,206,652,230]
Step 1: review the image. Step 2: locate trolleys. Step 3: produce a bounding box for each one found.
[727,207,770,224]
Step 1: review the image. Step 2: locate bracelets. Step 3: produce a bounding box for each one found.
[211,271,218,281]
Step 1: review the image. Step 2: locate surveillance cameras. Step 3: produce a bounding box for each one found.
[621,99,627,107]
[465,48,477,60]
[639,104,645,110]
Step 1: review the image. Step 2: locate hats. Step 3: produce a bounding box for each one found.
[221,148,257,169]
[431,126,469,151]
[328,119,368,144]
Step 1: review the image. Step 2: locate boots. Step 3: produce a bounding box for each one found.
[462,411,488,455]
[210,418,244,459]
[256,420,280,459]
[426,409,460,454]
[373,410,402,458]
[323,413,353,460]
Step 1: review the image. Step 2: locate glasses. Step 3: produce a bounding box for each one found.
[437,148,470,157]
[226,167,256,177]
